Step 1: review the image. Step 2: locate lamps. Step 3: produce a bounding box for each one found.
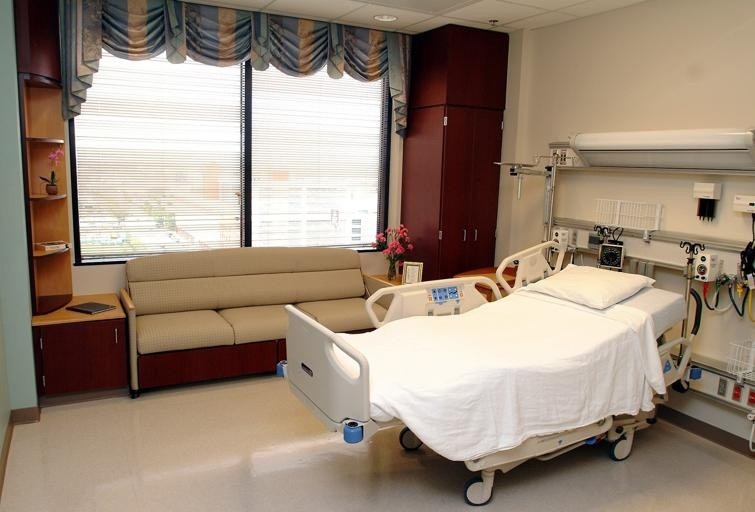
[568,128,755,176]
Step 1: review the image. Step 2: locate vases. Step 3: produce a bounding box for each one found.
[387,260,396,281]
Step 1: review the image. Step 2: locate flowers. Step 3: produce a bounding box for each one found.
[370,224,415,272]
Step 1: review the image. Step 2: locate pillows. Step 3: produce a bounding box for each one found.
[526,264,656,311]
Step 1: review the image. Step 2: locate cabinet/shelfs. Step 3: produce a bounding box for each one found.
[17,73,72,313]
[38,320,129,398]
[441,106,504,276]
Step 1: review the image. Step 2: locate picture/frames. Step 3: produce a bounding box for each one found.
[401,262,424,286]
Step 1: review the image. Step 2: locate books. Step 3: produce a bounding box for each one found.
[35,240,72,251]
[65,300,116,315]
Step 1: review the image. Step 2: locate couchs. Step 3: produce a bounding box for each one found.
[122,246,397,402]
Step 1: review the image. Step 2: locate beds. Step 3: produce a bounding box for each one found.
[278,240,704,504]
